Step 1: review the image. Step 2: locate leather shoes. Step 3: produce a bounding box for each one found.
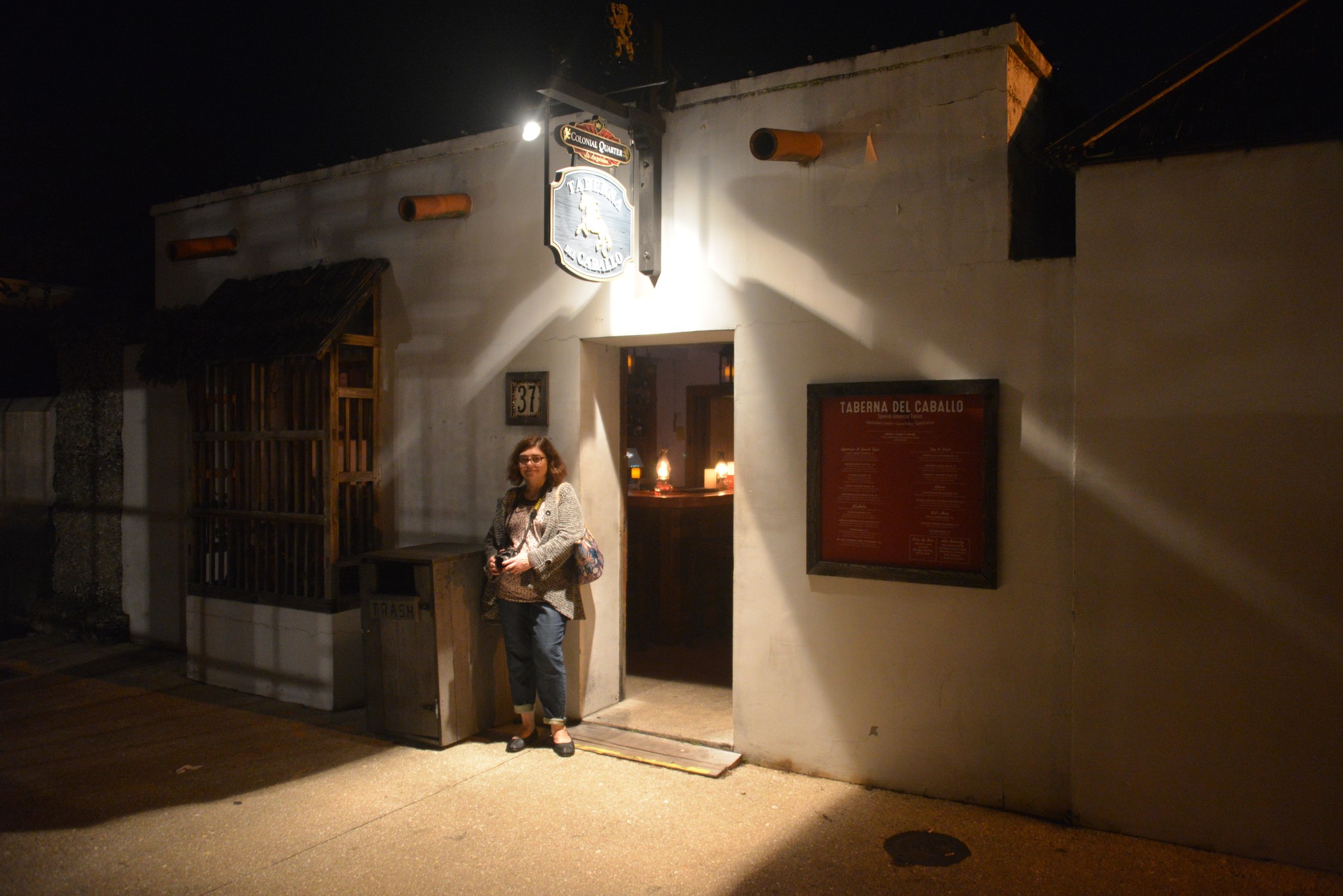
[506,728,538,752]
[550,730,575,756]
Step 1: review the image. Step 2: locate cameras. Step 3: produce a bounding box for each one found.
[495,548,518,570]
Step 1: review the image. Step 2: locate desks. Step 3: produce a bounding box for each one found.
[625,490,734,650]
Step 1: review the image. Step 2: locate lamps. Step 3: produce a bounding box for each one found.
[717,343,734,397]
[714,452,729,491]
[654,449,674,495]
[521,81,666,142]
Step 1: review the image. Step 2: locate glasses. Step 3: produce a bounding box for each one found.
[518,456,547,464]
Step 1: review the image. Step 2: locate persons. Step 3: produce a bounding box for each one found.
[478,434,605,758]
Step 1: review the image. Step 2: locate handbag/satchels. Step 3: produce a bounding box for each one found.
[557,482,604,585]
